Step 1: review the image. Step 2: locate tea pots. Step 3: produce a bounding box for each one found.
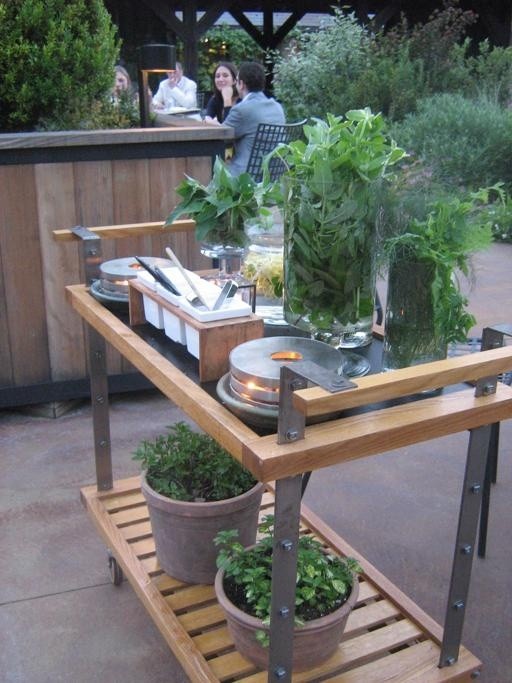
[211,278,241,317]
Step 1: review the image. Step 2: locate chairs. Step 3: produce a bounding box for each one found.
[196,90,205,115]
[242,115,309,187]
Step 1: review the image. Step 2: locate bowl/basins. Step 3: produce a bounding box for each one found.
[214,372,343,430]
[88,279,129,312]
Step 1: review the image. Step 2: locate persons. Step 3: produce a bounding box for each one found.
[152,61,198,112]
[107,64,152,113]
[213,62,288,187]
[201,63,241,124]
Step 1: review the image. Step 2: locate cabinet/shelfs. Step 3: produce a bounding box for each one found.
[57,216,512,682]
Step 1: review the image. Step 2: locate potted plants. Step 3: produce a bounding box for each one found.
[132,420,265,586]
[264,102,409,348]
[201,504,361,669]
[169,146,269,282]
[363,171,509,377]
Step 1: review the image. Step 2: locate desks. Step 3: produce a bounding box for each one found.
[155,105,201,117]
